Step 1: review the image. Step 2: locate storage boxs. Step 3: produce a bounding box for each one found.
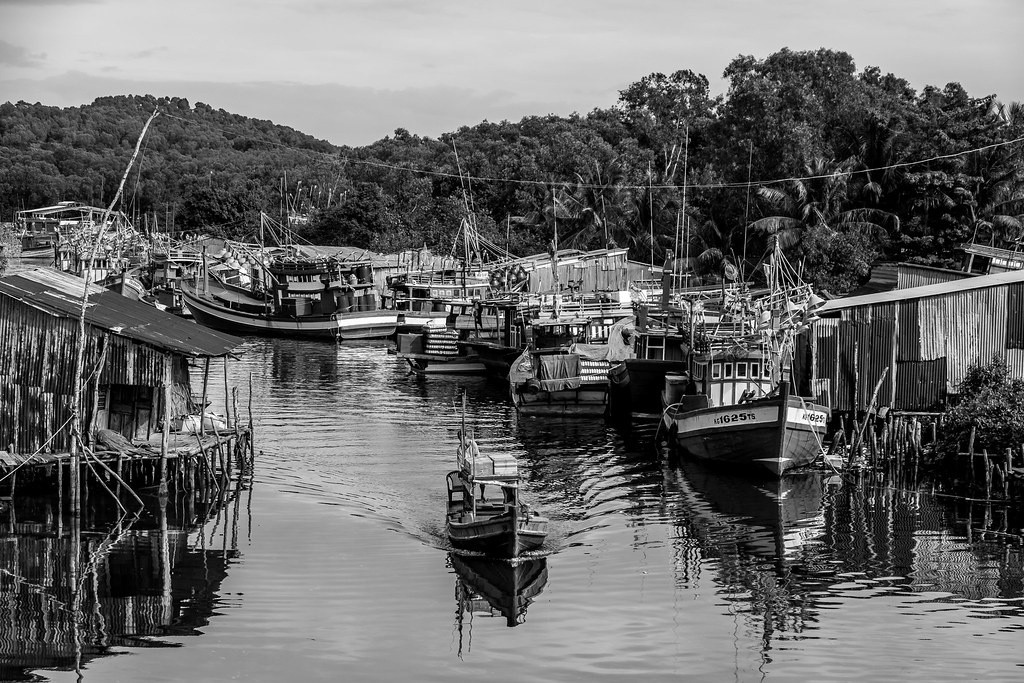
[465,453,518,476]
[665,375,688,405]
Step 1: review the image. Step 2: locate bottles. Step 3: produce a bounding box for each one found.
[461,511,474,523]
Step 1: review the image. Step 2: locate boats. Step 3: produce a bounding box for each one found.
[446,546,548,657]
[511,408,831,596]
[445,389,548,558]
[54,127,830,477]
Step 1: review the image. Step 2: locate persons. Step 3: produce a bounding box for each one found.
[457,431,480,512]
